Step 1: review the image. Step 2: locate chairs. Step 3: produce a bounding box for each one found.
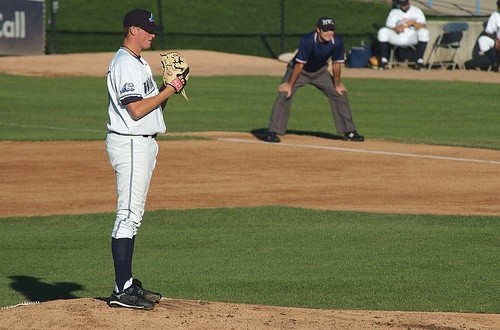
[372,24,417,70]
[425,23,469,72]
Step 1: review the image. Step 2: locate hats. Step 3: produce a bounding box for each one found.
[398,0,408,4]
[124,8,165,31]
[318,17,335,30]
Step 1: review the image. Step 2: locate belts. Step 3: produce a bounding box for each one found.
[144,134,157,137]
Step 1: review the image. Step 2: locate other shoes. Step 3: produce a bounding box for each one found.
[417,63,426,71]
[380,64,388,70]
[491,63,498,72]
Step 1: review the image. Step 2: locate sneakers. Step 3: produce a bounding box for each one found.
[267,132,280,142]
[344,131,364,141]
[109,287,155,310]
[132,279,162,302]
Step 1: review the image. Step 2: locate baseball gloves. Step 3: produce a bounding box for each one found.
[159,52,189,101]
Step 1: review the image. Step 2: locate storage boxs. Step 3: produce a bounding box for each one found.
[347,50,372,67]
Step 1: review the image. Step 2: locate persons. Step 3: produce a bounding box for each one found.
[104,9,192,309]
[478,0,500,71]
[376,0,430,72]
[266,17,365,142]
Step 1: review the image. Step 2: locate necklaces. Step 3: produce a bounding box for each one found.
[120,44,141,58]
